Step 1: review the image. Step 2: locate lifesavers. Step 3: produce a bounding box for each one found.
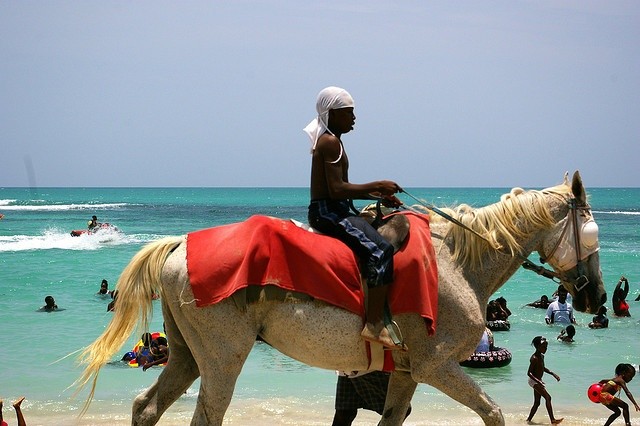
[129,331,169,367]
[487,319,510,331]
[460,346,512,367]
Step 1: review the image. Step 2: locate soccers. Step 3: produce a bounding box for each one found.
[587,384,604,403]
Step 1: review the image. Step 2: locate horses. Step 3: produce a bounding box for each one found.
[49,171,607,426]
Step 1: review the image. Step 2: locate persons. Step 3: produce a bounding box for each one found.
[34,296,66,313]
[597,363,640,426]
[97,279,113,295]
[87,215,98,231]
[107,290,121,312]
[557,325,576,343]
[0,397,28,425]
[545,285,577,325]
[612,276,632,317]
[528,295,552,309]
[475,326,494,352]
[526,336,564,425]
[121,332,169,372]
[303,85,410,352]
[588,306,609,329]
[333,372,412,426]
[487,297,511,321]
